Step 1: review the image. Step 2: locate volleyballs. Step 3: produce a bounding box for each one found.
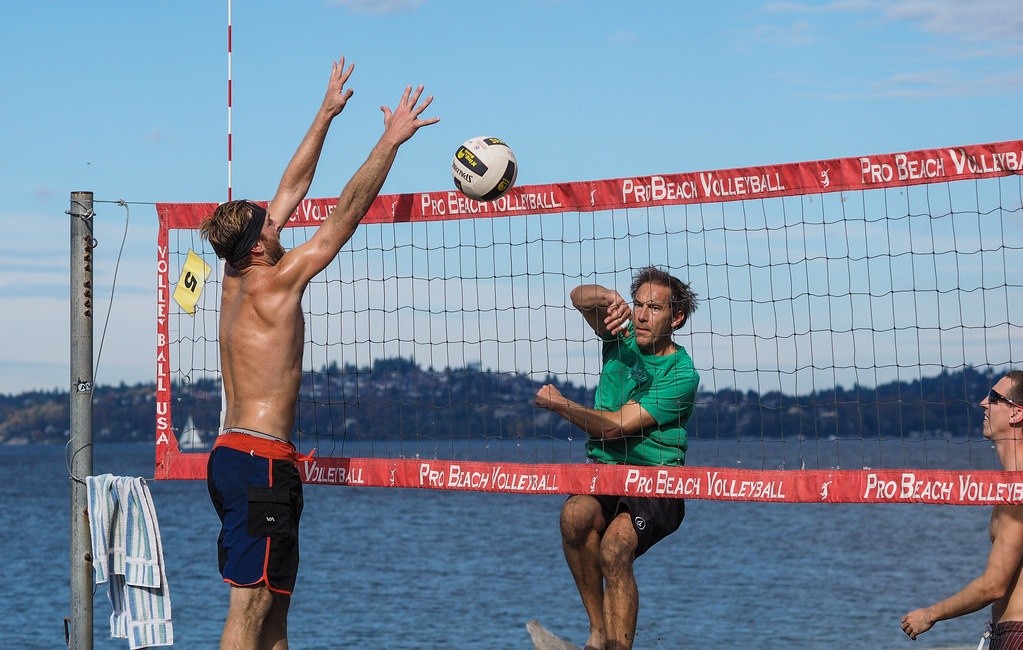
[450,133,519,199]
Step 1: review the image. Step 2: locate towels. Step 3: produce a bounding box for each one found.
[83,475,175,650]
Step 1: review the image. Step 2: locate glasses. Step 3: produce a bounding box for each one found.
[988,389,1023,409]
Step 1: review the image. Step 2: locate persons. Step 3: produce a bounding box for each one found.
[536,268,700,650]
[900,370,1023,649]
[200,57,441,650]
